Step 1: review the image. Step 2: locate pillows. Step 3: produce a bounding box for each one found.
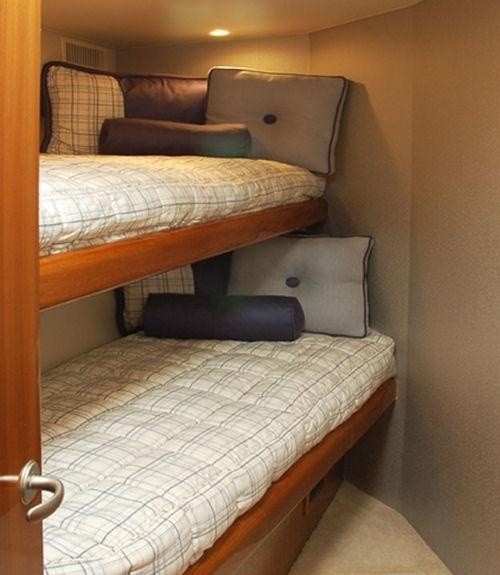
[40,62,211,156]
[204,61,348,170]
[226,232,373,340]
[99,117,253,158]
[145,293,304,342]
[118,262,197,338]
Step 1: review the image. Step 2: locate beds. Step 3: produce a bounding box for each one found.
[37,0,395,575]
